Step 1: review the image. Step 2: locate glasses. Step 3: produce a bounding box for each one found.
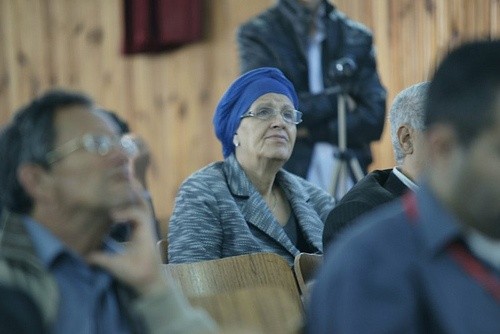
[240,106,303,124]
[41,132,150,165]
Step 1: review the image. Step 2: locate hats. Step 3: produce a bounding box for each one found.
[213,67,301,159]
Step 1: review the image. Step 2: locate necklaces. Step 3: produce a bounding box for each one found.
[270,191,278,210]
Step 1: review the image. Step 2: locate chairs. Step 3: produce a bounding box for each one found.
[165,251,323,334]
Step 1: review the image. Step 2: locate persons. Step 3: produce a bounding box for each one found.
[0,37,500,334]
[234,0,388,203]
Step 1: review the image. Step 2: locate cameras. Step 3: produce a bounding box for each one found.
[329,56,358,85]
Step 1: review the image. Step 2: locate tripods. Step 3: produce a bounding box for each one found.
[330,85,365,200]
